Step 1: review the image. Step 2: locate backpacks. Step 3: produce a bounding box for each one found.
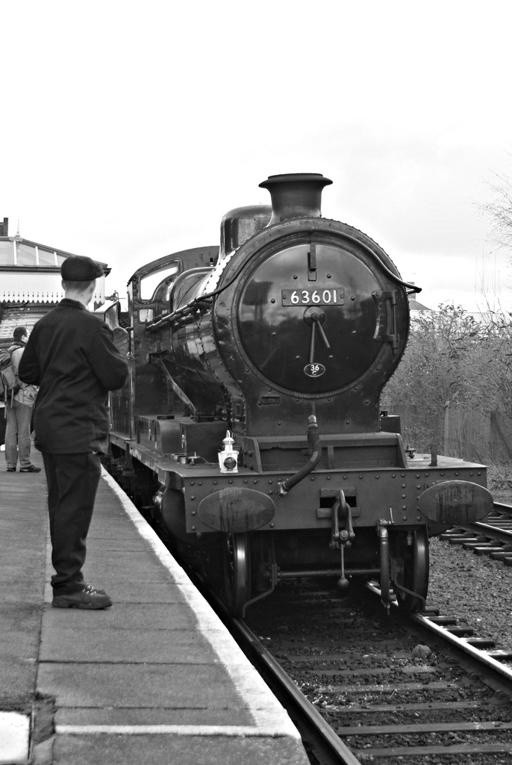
[0,346,30,400]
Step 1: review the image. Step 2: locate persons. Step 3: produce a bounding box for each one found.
[5,327,40,473]
[18,256,130,611]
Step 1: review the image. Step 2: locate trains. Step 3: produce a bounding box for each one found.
[105,171,493,617]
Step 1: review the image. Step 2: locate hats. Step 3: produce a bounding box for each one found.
[61,256,104,282]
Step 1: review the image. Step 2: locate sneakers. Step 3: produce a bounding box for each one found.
[52,584,113,611]
[7,467,17,473]
[20,465,42,473]
